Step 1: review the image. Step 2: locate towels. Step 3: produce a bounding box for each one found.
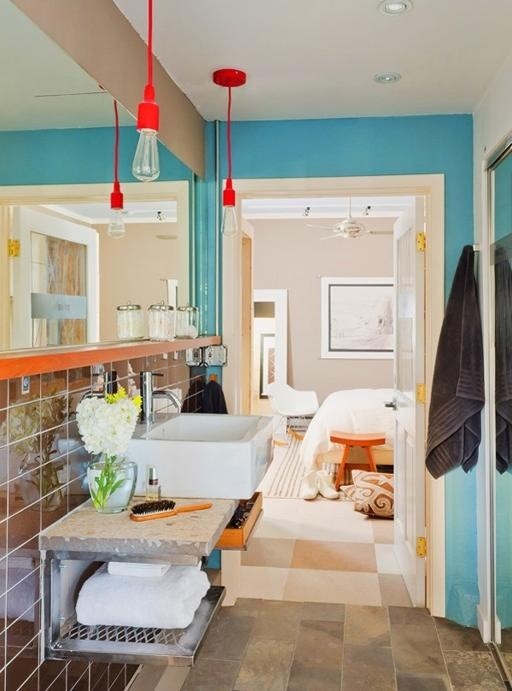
[75,560,210,629]
[0,547,38,622]
[0,547,39,570]
[107,560,170,577]
[426,245,485,480]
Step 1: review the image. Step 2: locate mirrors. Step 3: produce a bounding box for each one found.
[251,288,288,443]
[0,0,209,370]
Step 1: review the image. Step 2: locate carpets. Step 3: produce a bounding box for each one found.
[265,430,394,500]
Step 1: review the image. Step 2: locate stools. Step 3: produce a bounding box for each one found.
[329,429,385,488]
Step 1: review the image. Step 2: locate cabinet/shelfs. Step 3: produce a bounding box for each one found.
[39,496,240,666]
[0,493,39,658]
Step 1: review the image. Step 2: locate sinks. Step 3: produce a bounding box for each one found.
[34,415,103,494]
[128,413,273,500]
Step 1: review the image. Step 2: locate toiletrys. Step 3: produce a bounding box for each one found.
[145,468,161,503]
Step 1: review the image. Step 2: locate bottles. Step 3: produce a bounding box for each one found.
[175,301,199,341]
[116,299,144,341]
[146,298,174,343]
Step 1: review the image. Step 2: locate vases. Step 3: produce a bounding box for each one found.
[18,456,72,511]
[87,461,138,513]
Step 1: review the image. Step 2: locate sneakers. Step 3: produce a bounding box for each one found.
[299,470,340,500]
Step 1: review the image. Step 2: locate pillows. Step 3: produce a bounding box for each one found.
[340,470,394,519]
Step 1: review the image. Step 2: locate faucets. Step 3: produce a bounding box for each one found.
[139,371,182,424]
[79,370,118,402]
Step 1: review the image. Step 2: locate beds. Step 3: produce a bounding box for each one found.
[299,388,395,468]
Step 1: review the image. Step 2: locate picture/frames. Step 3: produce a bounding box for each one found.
[254,318,276,401]
[321,277,395,360]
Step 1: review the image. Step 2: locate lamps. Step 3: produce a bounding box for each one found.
[212,68,247,237]
[108,99,126,238]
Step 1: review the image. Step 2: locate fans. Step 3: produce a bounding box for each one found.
[306,198,395,241]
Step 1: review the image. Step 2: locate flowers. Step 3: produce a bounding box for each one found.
[77,388,142,507]
[1,390,75,508]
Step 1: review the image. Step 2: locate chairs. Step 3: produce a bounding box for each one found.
[267,381,318,441]
